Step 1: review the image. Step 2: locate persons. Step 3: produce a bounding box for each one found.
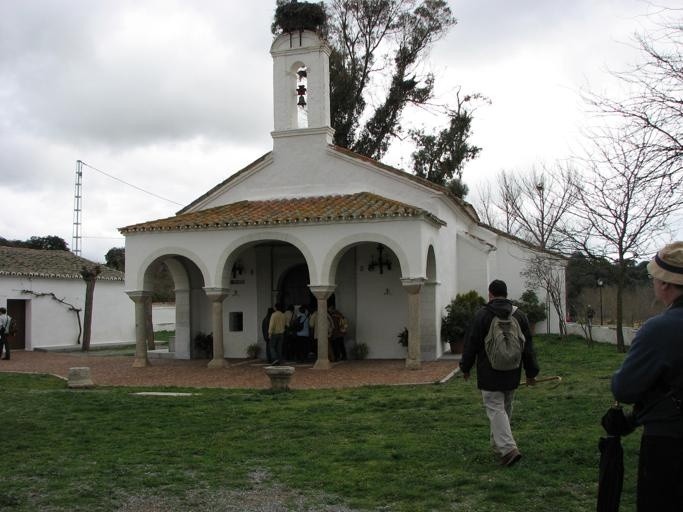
[457,278,540,468]
[569,304,577,322]
[610,240,682,511]
[585,304,595,330]
[0,307,13,361]
[259,300,350,366]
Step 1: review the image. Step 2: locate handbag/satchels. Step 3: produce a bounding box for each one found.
[0,326,6,336]
[9,318,18,337]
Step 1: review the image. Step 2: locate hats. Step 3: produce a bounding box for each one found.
[647,241,683,285]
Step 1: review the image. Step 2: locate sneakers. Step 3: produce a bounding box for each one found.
[502,449,521,467]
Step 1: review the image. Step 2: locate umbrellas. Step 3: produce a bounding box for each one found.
[597,398,630,511]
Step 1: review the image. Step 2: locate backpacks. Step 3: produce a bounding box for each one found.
[481,305,526,371]
[289,315,302,334]
[333,314,350,333]
